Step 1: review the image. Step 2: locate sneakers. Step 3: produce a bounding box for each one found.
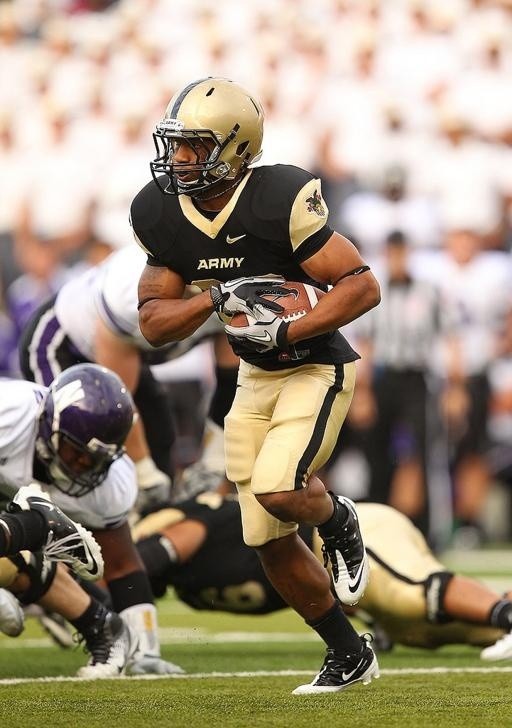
[39,609,75,650]
[291,632,380,696]
[5,482,105,584]
[76,609,139,680]
[479,632,512,661]
[321,496,369,608]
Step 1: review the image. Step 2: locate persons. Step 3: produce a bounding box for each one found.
[1,77,511,697]
[1,0,510,549]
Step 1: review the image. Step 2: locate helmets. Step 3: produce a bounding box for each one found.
[150,75,264,203]
[33,363,133,497]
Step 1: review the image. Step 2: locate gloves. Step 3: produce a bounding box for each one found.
[135,457,172,512]
[225,303,290,358]
[119,604,186,674]
[210,274,289,319]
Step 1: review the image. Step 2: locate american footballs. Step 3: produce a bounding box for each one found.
[229,281,328,343]
